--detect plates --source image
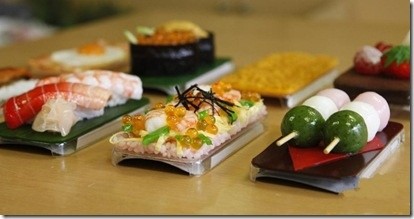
[143,58,236,98]
[249,120,404,178]
[332,66,410,105]
[0,96,150,156]
[263,68,338,110]
[109,116,266,175]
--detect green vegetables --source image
[381,44,410,67]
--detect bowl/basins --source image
[130,31,215,75]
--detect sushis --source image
[0,68,143,137]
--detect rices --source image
[110,96,267,161]
